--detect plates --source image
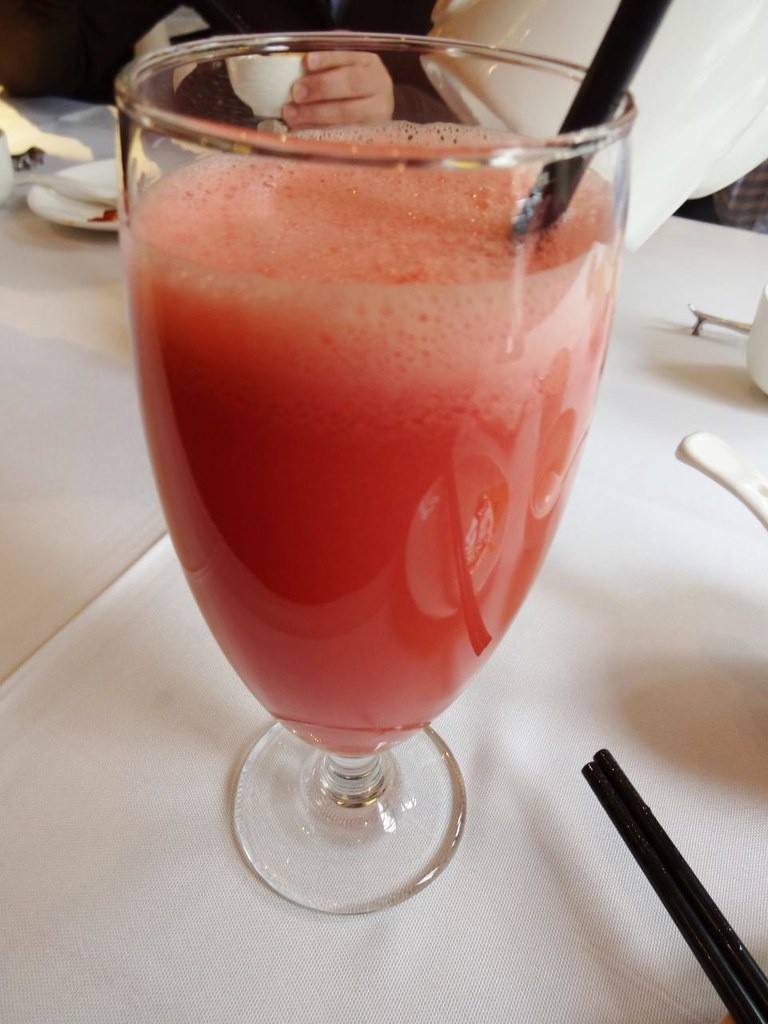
[27,161,118,230]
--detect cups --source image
[745,281,768,395]
[0,128,17,204]
[225,52,306,119]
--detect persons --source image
[0,0,459,131]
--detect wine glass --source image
[117,28,639,917]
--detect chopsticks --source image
[580,747,768,1024]
